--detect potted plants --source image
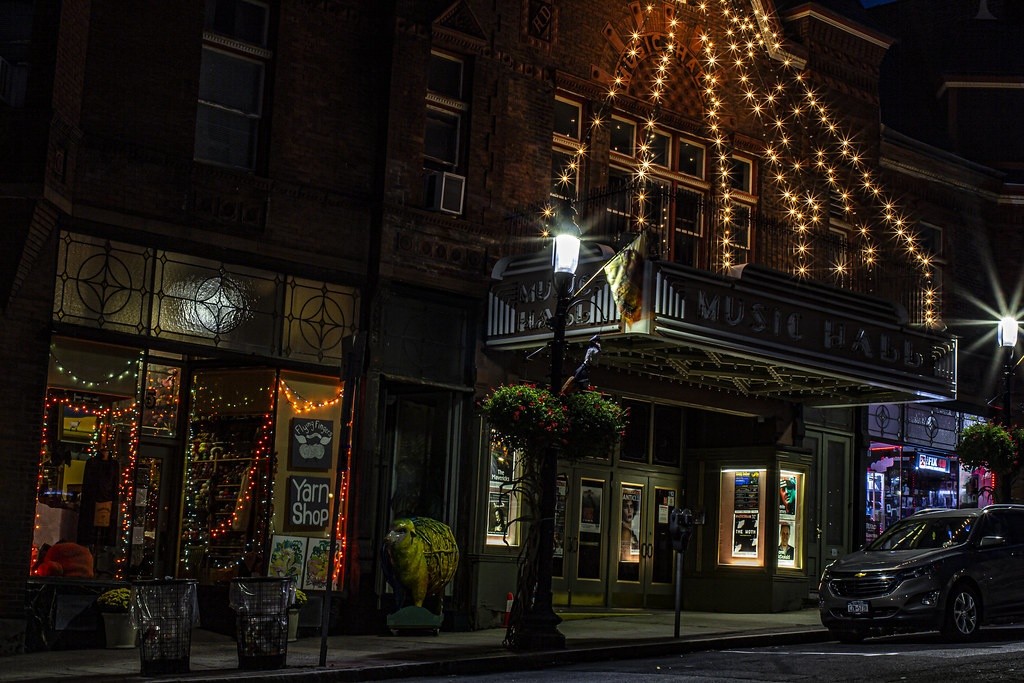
[96,588,139,648]
[289,586,308,642]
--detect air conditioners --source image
[423,171,467,215]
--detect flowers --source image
[956,415,1019,476]
[560,387,633,464]
[473,380,569,455]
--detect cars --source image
[816,503,1024,645]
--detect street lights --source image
[512,190,584,651]
[997,311,1019,504]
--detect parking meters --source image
[667,506,698,640]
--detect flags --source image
[603,234,657,325]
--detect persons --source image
[779,479,795,515]
[620,489,640,561]
[778,522,794,560]
[32,538,67,571]
[490,440,513,484]
[489,507,507,532]
[553,531,564,553]
[583,490,597,523]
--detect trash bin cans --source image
[229,576,291,669]
[132,579,200,676]
